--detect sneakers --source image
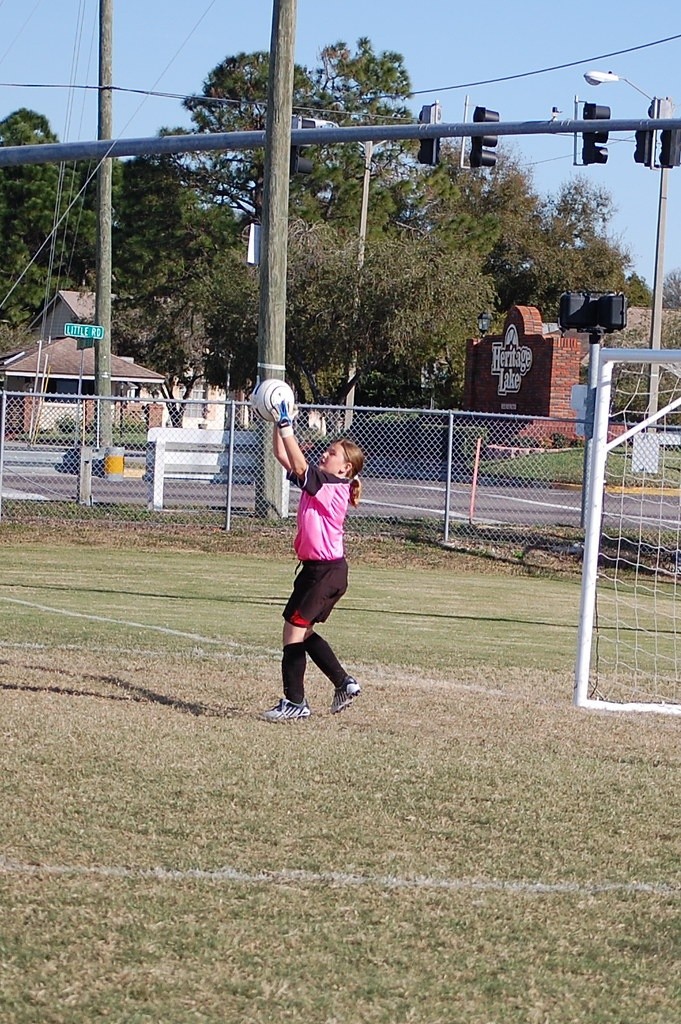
[263,697,310,719]
[331,676,361,715]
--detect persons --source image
[261,400,364,721]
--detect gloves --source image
[270,400,299,438]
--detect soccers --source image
[249,378,296,422]
